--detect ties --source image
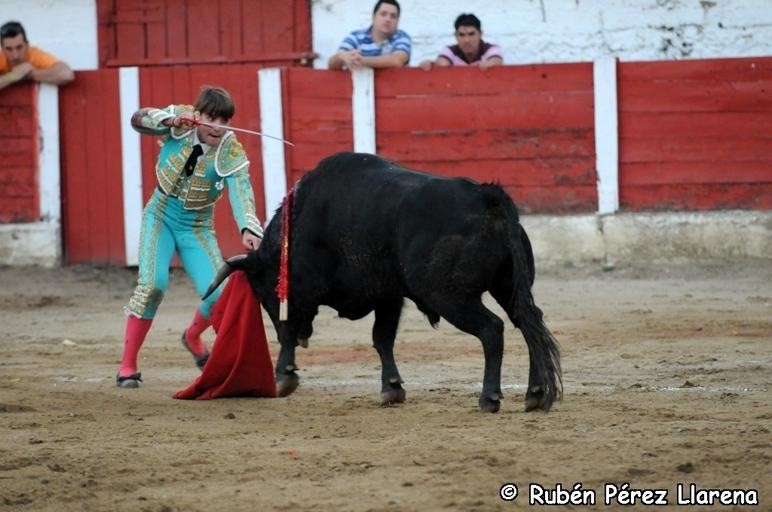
[183,144,206,177]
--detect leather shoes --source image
[180,327,210,372]
[116,369,144,389]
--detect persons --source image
[0,20,77,89]
[329,0,412,71]
[114,86,266,389]
[420,13,503,72]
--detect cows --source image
[200,151,563,415]
